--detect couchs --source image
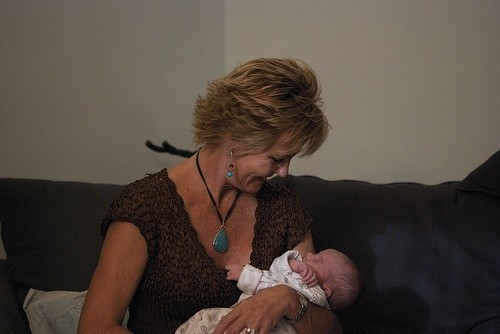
[0,174,500,334]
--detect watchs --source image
[285,292,308,324]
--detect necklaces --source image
[196,147,241,252]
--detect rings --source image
[246,327,255,334]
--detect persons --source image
[77,58,343,334]
[175,249,361,334]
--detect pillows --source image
[457,149,500,199]
[23,288,129,334]
